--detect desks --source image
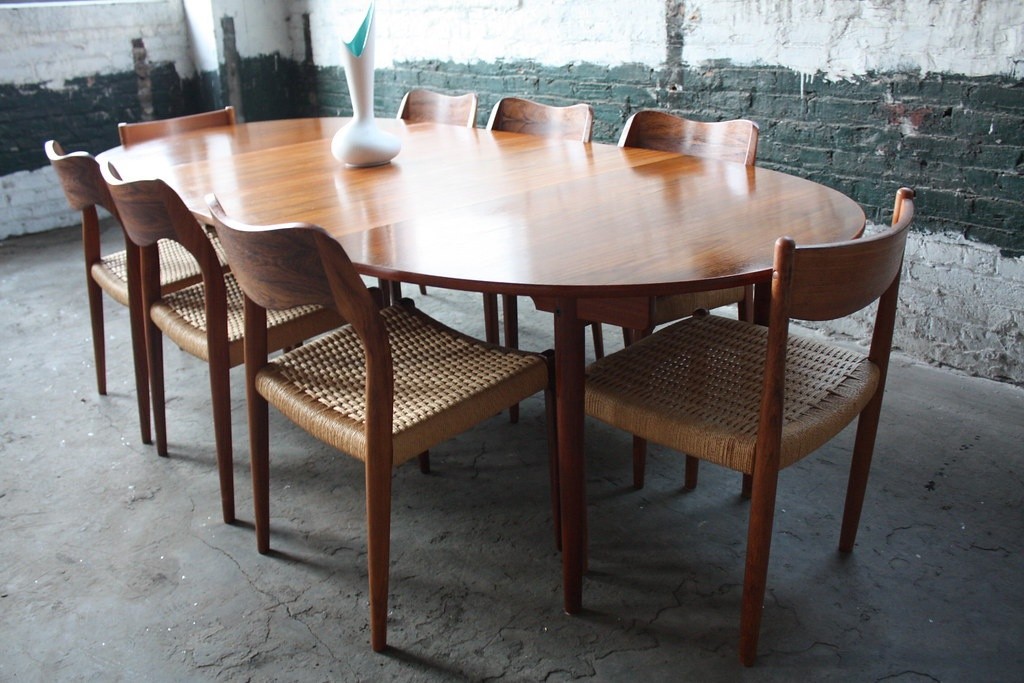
[93,116,868,620]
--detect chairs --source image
[101,160,387,525]
[502,109,759,424]
[397,89,480,129]
[206,191,557,653]
[118,105,236,149]
[44,140,232,446]
[442,96,605,424]
[557,186,918,671]
